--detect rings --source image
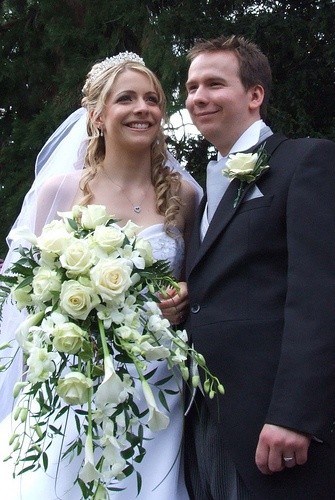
[283,457,294,462]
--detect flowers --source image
[0,203,225,500]
[221,141,270,208]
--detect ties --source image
[206,126,274,224]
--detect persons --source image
[184,34,335,500]
[0,50,203,500]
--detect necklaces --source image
[99,162,152,213]
